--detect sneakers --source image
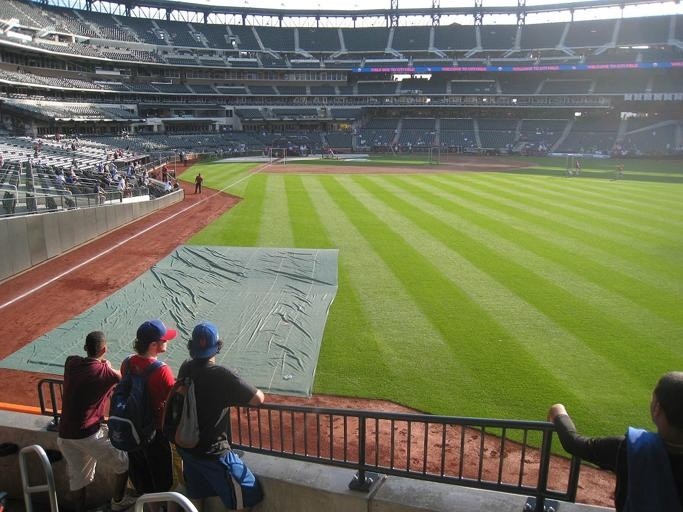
[111,492,139,511]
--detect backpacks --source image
[107,357,165,454]
[162,363,213,449]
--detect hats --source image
[136,318,177,342]
[189,323,220,361]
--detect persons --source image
[173,322,265,510]
[26,117,247,206]
[548,370,683,510]
[55,330,131,509]
[116,319,179,509]
